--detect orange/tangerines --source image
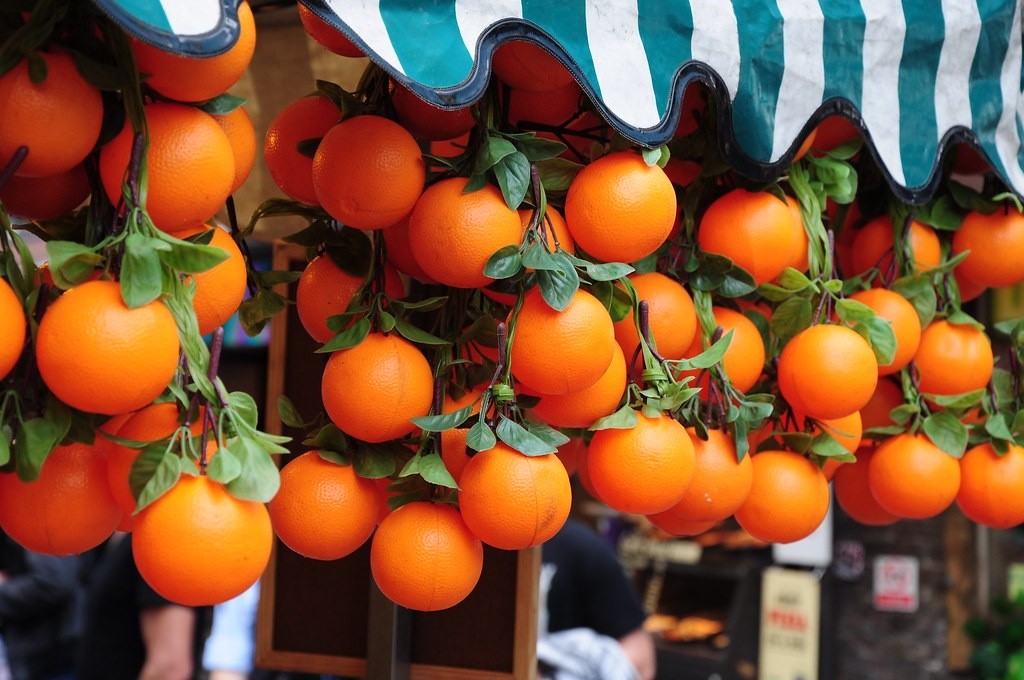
[0,2,1024,612]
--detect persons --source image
[0,530,259,680]
[538,516,656,680]
